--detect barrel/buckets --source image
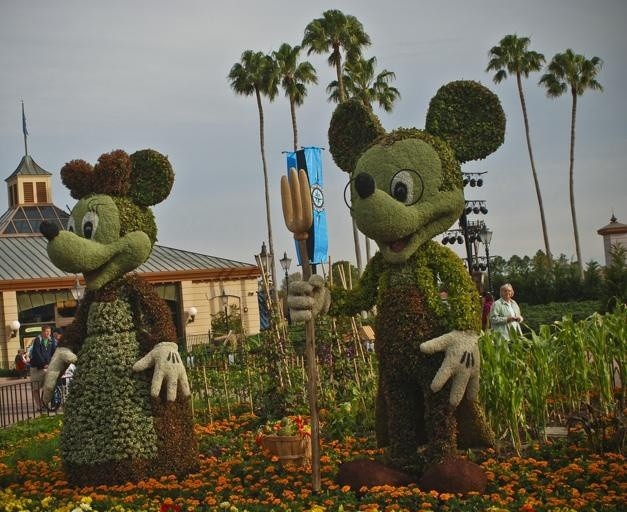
[260,436,276,456]
[276,437,306,469]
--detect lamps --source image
[188,307,196,322]
[10,321,20,337]
[442,173,487,273]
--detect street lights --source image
[279,250,292,326]
[69,273,86,310]
[439,171,493,290]
[479,222,494,301]
[219,290,229,336]
[253,240,276,330]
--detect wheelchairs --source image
[38,361,73,412]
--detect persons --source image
[481,281,524,346]
[14,326,76,412]
